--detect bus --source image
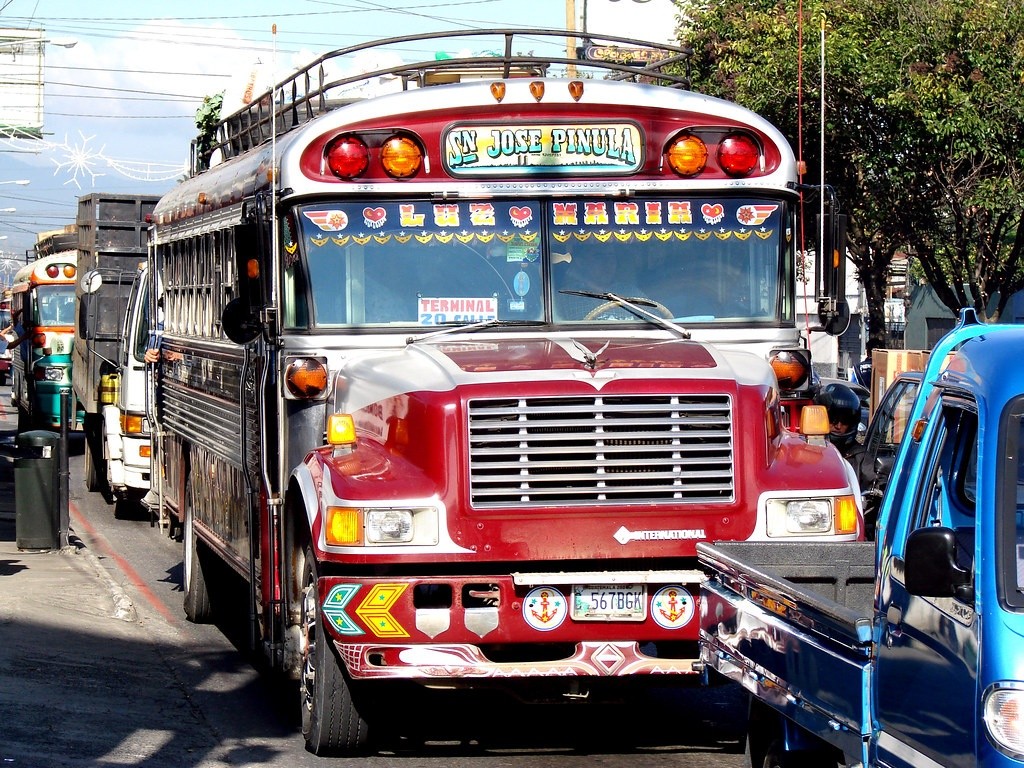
[140,21,872,757]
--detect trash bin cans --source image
[0,429,61,551]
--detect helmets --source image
[800,376,820,399]
[817,383,861,445]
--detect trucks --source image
[690,307,1024,768]
[73,191,159,521]
[0,225,86,448]
[791,250,909,339]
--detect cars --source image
[847,372,1024,540]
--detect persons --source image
[855,338,886,388]
[0,308,34,401]
[814,383,888,542]
[140,292,166,510]
[555,242,661,321]
